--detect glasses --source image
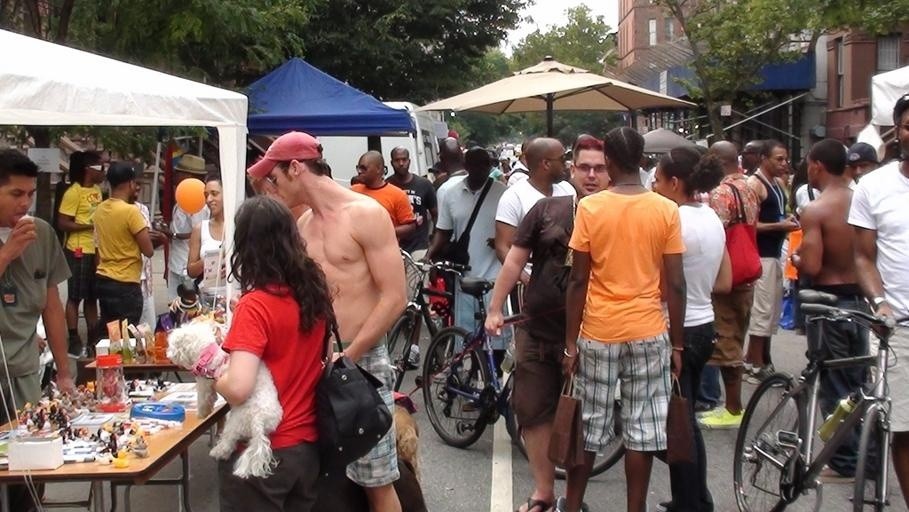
[573,161,609,175]
[355,162,378,171]
[898,122,909,131]
[86,164,106,174]
[549,155,568,164]
[766,155,789,163]
[740,149,758,156]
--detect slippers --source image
[515,493,558,512]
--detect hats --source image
[245,130,327,184]
[845,140,881,167]
[106,159,145,186]
[891,93,909,120]
[171,151,212,177]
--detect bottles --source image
[818,391,860,441]
[500,341,516,373]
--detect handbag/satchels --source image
[654,370,700,470]
[720,180,765,292]
[429,236,473,276]
[313,281,398,469]
[544,369,588,471]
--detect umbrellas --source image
[409,55,699,136]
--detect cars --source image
[501,140,521,156]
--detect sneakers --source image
[551,497,592,512]
[696,404,749,431]
[741,360,754,383]
[814,462,857,485]
[403,343,423,370]
[746,361,795,388]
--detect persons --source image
[0,94,908,510]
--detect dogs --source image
[165,319,284,480]
[394,403,422,487]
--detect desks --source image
[84,327,220,449]
[0,377,229,511]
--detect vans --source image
[307,101,451,204]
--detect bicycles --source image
[733,286,909,512]
[384,246,471,401]
[413,270,627,481]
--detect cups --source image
[94,354,129,413]
[196,375,217,418]
